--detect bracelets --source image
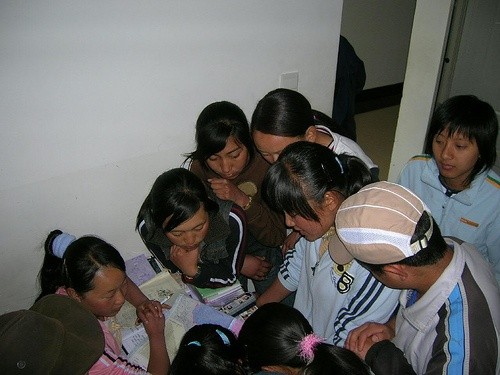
[241,195,253,209]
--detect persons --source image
[250,88,381,183]
[238,302,375,375]
[180,100,296,307]
[335,181,500,375]
[331,35,366,144]
[394,95,500,284]
[28,230,171,375]
[169,324,249,375]
[135,168,248,289]
[253,140,405,347]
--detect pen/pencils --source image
[135,293,174,326]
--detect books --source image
[115,268,259,370]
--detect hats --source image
[1,295,105,375]
[328,181,433,265]
